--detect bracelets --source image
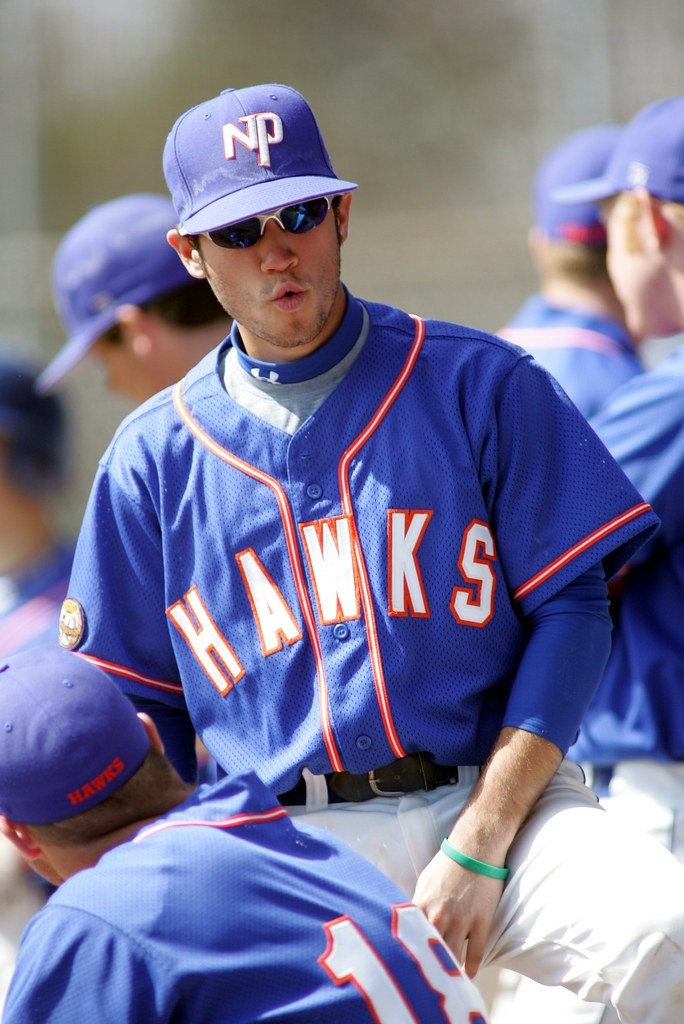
[441,838,508,878]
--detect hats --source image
[161,83,357,235]
[35,193,204,397]
[0,368,63,450]
[0,644,149,825]
[533,123,629,248]
[552,97,684,205]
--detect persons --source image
[61,82,683,1024]
[0,104,684,1024]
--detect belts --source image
[277,750,481,808]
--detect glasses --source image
[178,194,337,249]
